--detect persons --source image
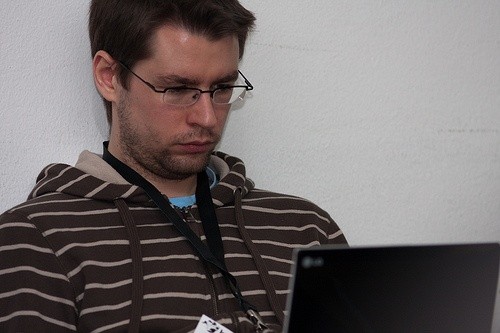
[0,0,351,333]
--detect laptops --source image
[282,242,500,333]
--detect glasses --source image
[115,60,254,106]
